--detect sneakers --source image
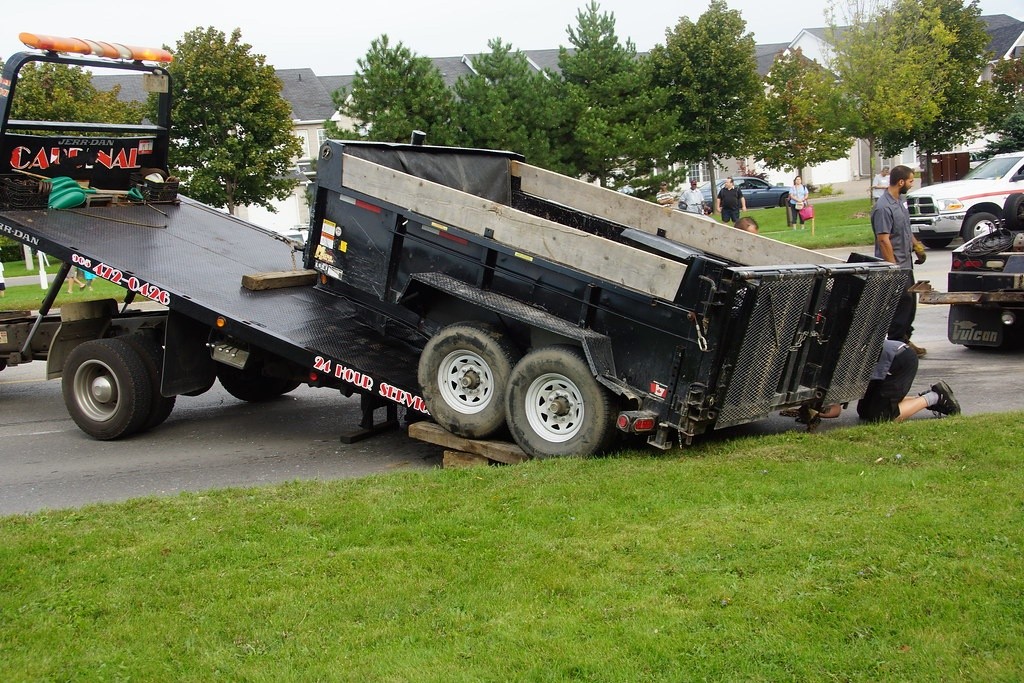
[918,389,949,417]
[929,380,961,415]
[907,340,927,356]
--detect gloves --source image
[912,241,926,264]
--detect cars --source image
[697,177,792,212]
[906,151,1024,250]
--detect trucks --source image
[905,192,1024,351]
[0,32,430,440]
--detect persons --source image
[786,176,809,230]
[656,180,712,216]
[734,217,758,235]
[819,340,961,422]
[65,265,99,293]
[0,261,6,298]
[716,177,746,225]
[871,165,926,357]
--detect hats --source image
[660,181,667,186]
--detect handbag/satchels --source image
[799,199,814,221]
[795,200,804,210]
